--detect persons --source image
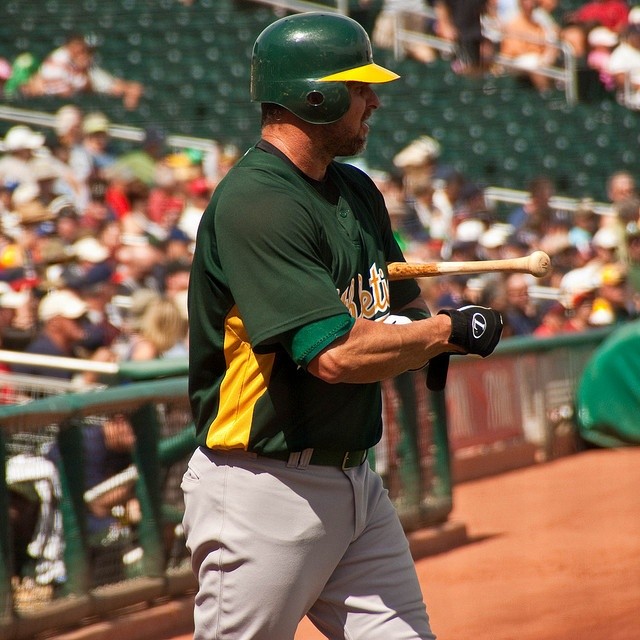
[370,0,640,110]
[374,135,638,344]
[31,32,142,108]
[180,11,505,640]
[0,106,243,395]
[44,413,142,552]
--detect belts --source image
[260,448,370,468]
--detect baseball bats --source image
[384,250,550,281]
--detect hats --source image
[68,26,100,47]
[0,280,30,308]
[75,236,112,263]
[144,127,170,146]
[82,111,109,135]
[3,125,46,152]
[457,219,485,242]
[588,26,620,46]
[188,178,212,196]
[541,233,578,258]
[61,259,117,285]
[38,288,87,321]
[592,229,619,248]
[16,202,58,223]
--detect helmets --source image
[251,12,401,124]
[559,269,601,307]
